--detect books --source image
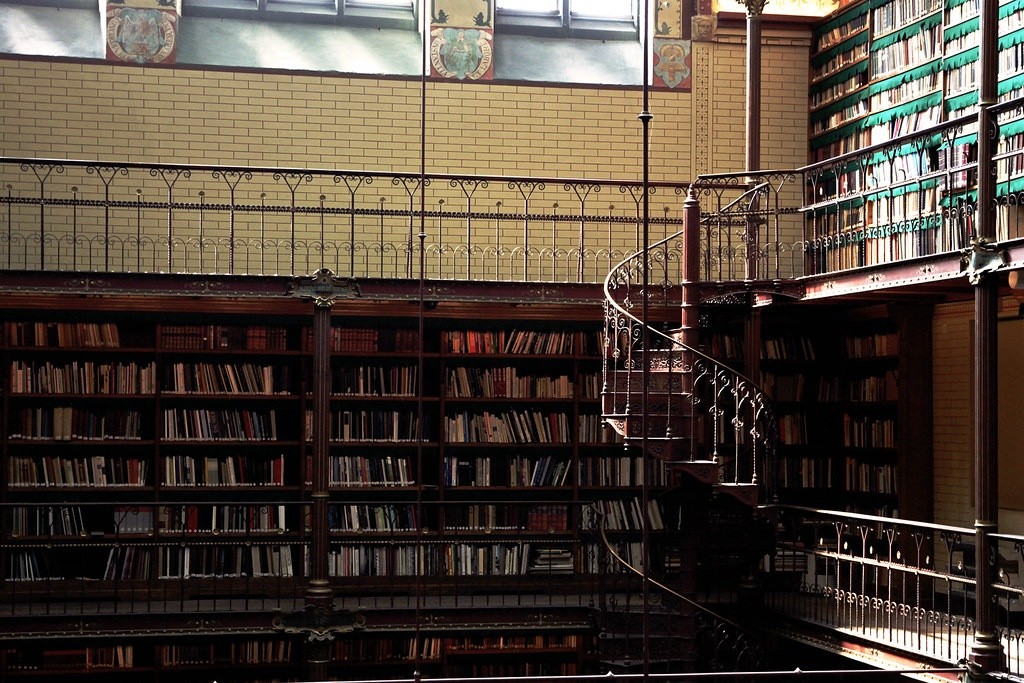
[812,0,1024,272]
[0,322,900,683]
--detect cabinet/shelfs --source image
[801,0,1024,274]
[0,293,937,683]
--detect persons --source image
[694,17,713,40]
